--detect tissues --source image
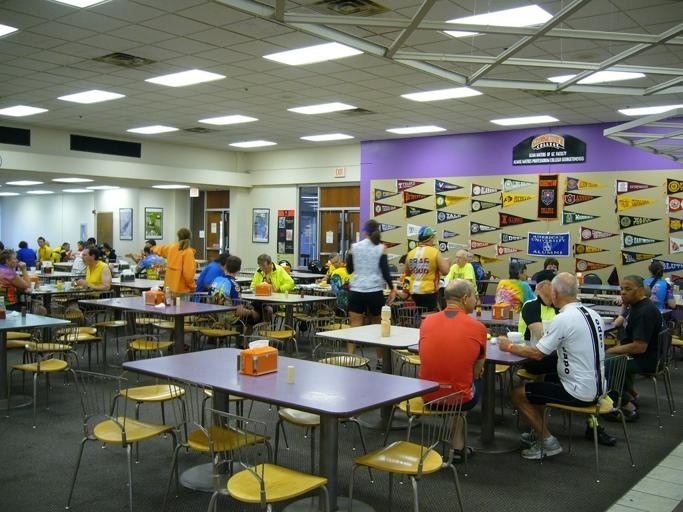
[576,272,584,285]
[255,282,272,296]
[145,286,165,305]
[665,278,674,287]
[240,340,279,376]
[27,275,40,289]
[491,303,511,320]
[40,261,54,273]
[120,269,135,282]
[118,259,129,270]
[280,262,290,274]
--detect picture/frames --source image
[119,208,133,240]
[145,207,163,240]
[252,208,270,243]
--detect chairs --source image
[100,335,190,465]
[65,368,180,511]
[2,260,683,430]
[537,355,636,485]
[164,374,274,512]
[202,404,331,511]
[346,389,466,512]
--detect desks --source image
[121,346,441,511]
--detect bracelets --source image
[508,344,513,353]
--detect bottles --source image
[283,289,305,298]
[380,303,391,337]
[286,365,295,383]
[476,299,481,318]
[0,296,6,319]
[165,287,171,305]
[175,296,180,306]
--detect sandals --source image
[453,446,476,464]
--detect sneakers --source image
[520,429,538,444]
[600,409,637,423]
[520,437,562,460]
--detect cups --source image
[673,295,682,303]
[27,260,80,289]
[321,280,326,287]
[506,331,522,343]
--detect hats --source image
[536,270,556,284]
[418,227,436,241]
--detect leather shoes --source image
[586,425,617,446]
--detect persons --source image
[517,271,639,447]
[190,253,260,348]
[418,278,487,463]
[531,258,559,292]
[1,236,117,313]
[498,271,607,461]
[494,262,537,314]
[605,261,677,414]
[314,251,354,332]
[135,240,166,280]
[250,253,295,327]
[150,228,198,294]
[345,219,397,370]
[396,225,485,313]
